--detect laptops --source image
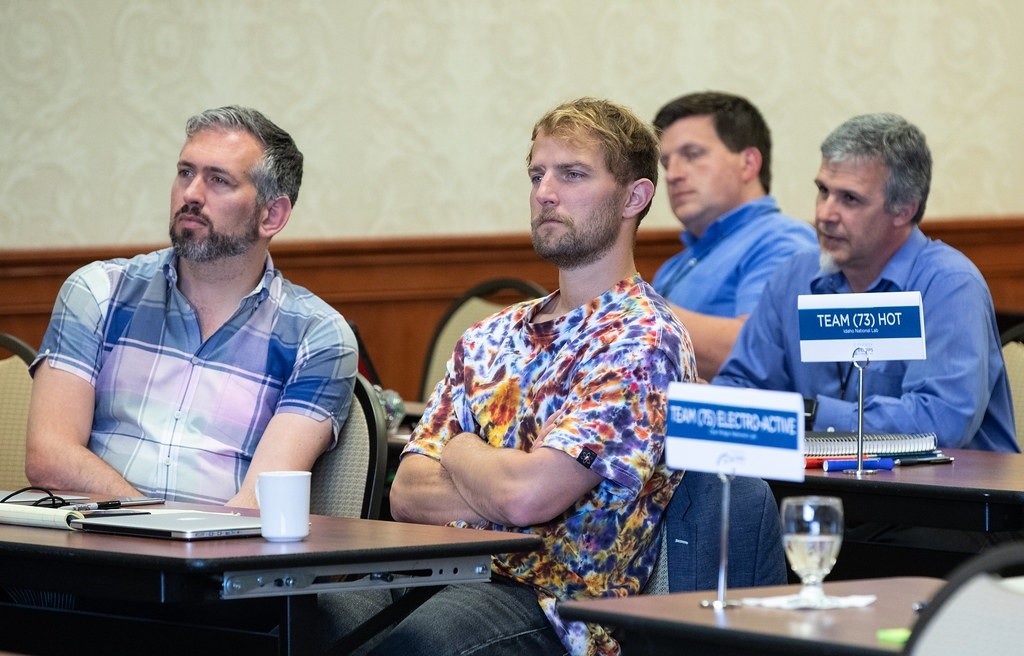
[68,510,263,541]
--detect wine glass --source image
[778,495,842,607]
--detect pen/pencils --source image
[911,600,928,613]
[73,500,121,511]
[894,457,955,466]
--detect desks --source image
[768,447,1024,582]
[0,489,546,656]
[557,577,947,656]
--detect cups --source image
[254,471,311,543]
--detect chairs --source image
[1001,313,1024,452]
[309,372,388,520]
[416,275,546,401]
[0,332,38,492]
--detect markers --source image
[805,455,869,469]
[823,458,894,471]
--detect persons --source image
[650,90,818,389]
[23,104,359,510]
[291,96,699,656]
[709,112,1021,455]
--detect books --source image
[803,431,938,457]
